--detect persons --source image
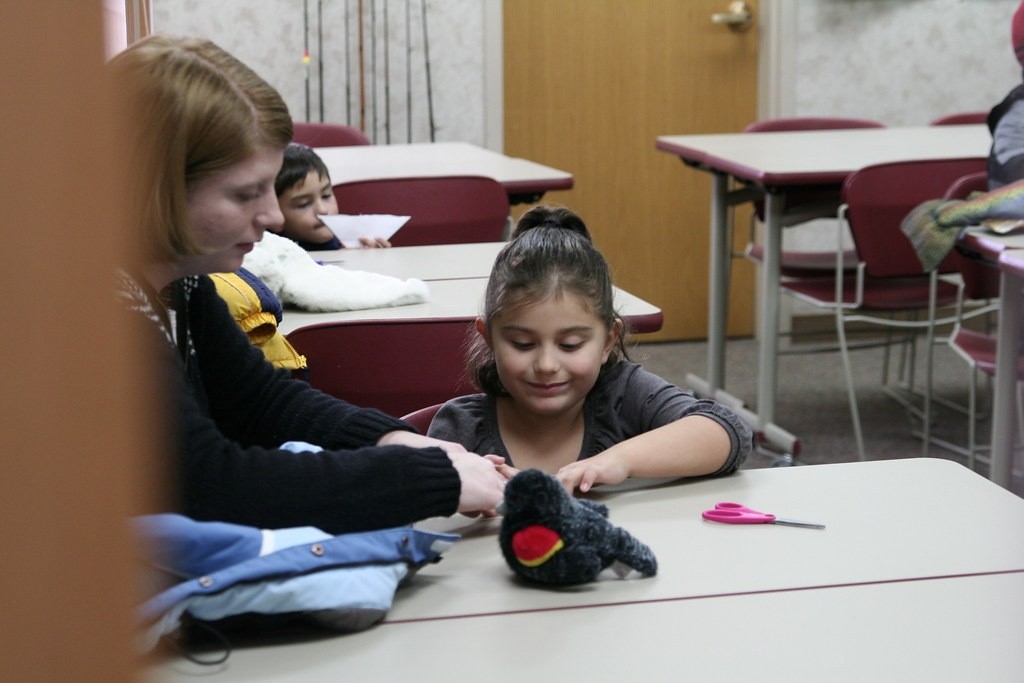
[427,206,753,497]
[986,1,1024,195]
[102,30,509,534]
[274,143,392,252]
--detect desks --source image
[124,113,1023,682]
[310,139,576,206]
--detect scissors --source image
[701,501,826,529]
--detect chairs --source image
[329,177,517,252]
[779,154,991,462]
[915,173,1024,482]
[285,316,481,420]
[750,117,889,286]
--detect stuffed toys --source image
[498,469,658,589]
